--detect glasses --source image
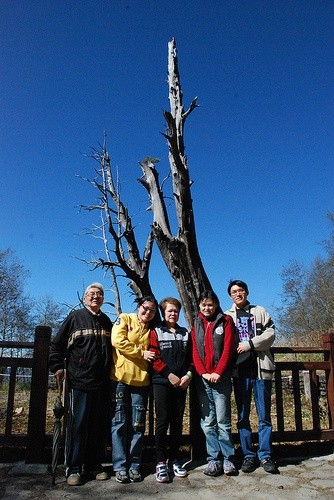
[141,305,156,313]
[85,292,103,299]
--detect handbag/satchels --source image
[236,351,258,378]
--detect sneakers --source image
[155,461,169,483]
[165,458,187,477]
[114,470,141,484]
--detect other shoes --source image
[202,460,222,476]
[67,473,82,486]
[85,469,110,480]
[260,457,274,472]
[241,457,258,473]
[223,459,237,474]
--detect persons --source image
[222,279,278,473]
[47,281,112,485]
[188,290,238,476]
[148,297,192,483]
[111,297,158,484]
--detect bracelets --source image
[186,374,192,380]
